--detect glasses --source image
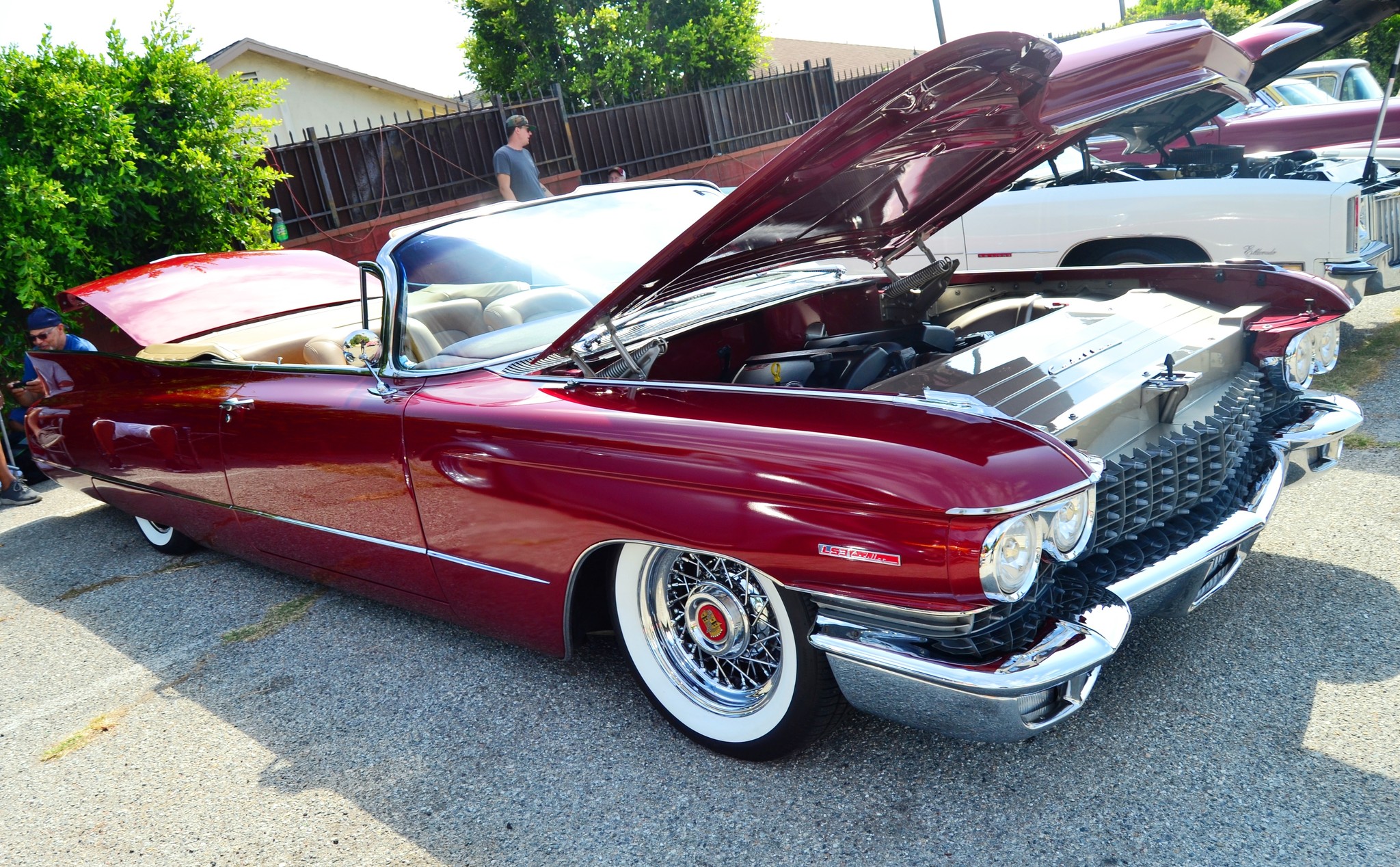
[519,127,530,132]
[29,327,56,341]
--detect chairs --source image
[302,284,595,369]
[96,418,210,469]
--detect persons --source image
[0,392,43,505]
[606,167,626,182]
[7,308,97,433]
[493,115,554,202]
[359,337,366,354]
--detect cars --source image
[885,0,1400,309]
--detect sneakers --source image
[0,478,44,505]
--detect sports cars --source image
[21,27,1363,764]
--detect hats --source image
[506,115,537,131]
[28,307,70,330]
[608,166,626,179]
